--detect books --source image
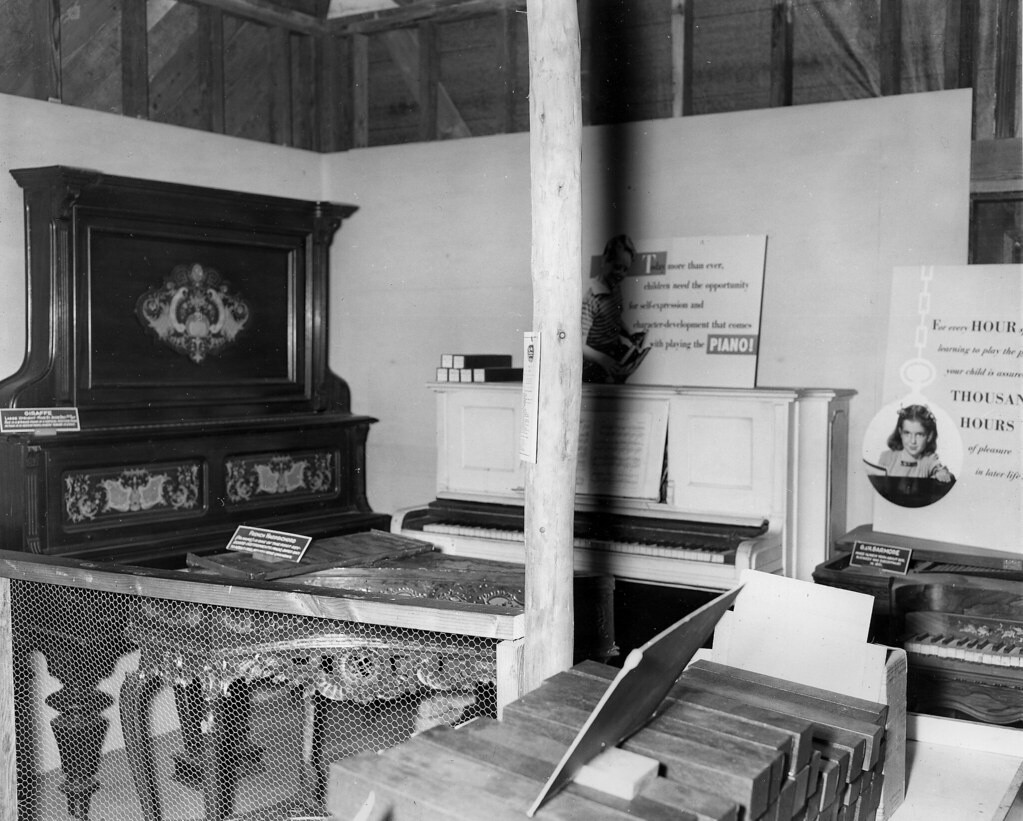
[578,395,670,502]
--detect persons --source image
[580,233,637,384]
[878,402,954,484]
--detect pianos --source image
[811,520,1023,729]
[387,377,859,599]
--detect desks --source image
[118,556,619,821]
